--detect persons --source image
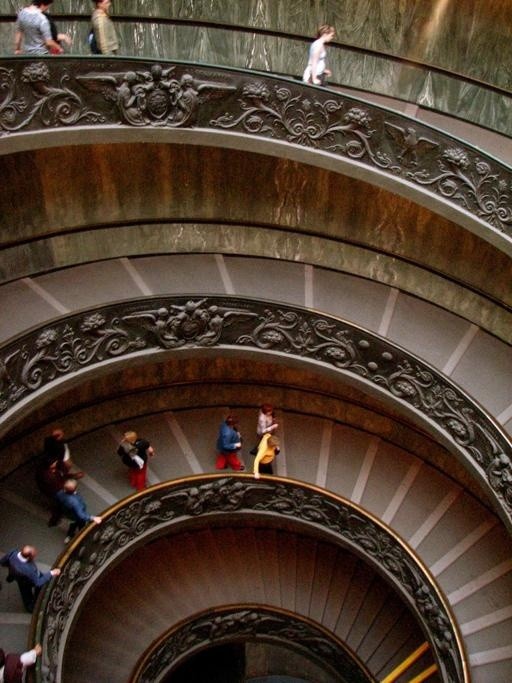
[14,0,66,54]
[249,402,281,456]
[302,24,335,88]
[33,449,85,527]
[215,414,245,470]
[43,429,85,478]
[57,478,103,545]
[1,545,62,614]
[252,433,282,479]
[31,0,76,53]
[91,0,121,55]
[0,641,43,683]
[116,430,156,491]
